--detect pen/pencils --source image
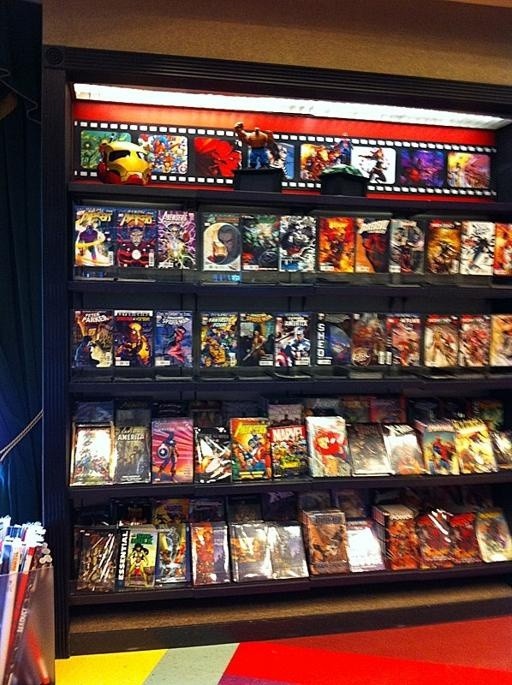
[0,517,56,685]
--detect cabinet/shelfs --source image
[41,49,511,657]
